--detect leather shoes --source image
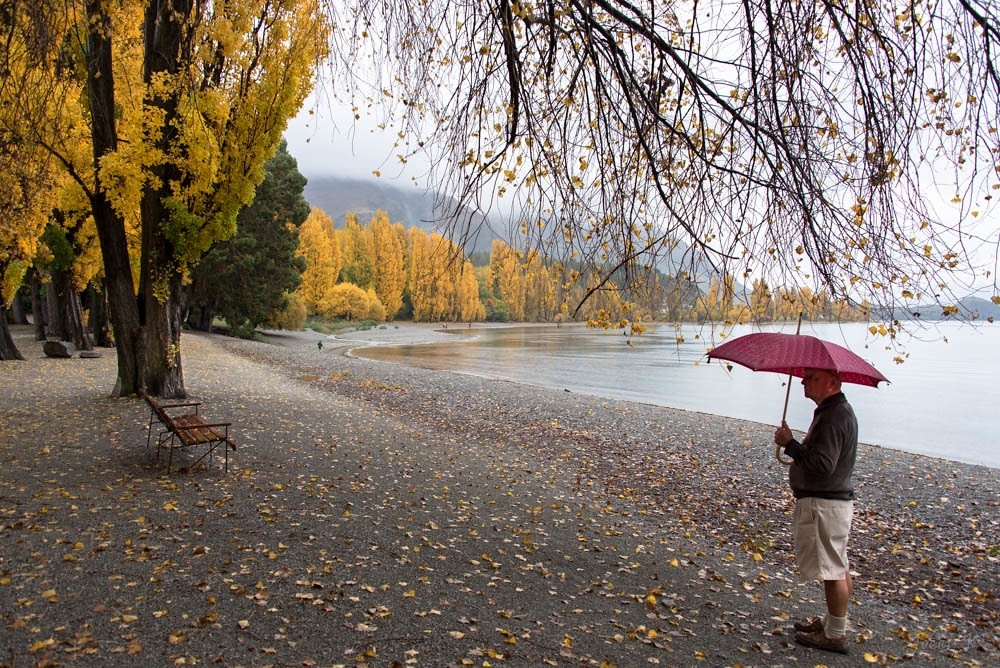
[795,629,849,655]
[794,617,824,633]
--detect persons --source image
[774,365,860,655]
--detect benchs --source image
[139,388,238,475]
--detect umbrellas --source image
[703,311,892,465]
